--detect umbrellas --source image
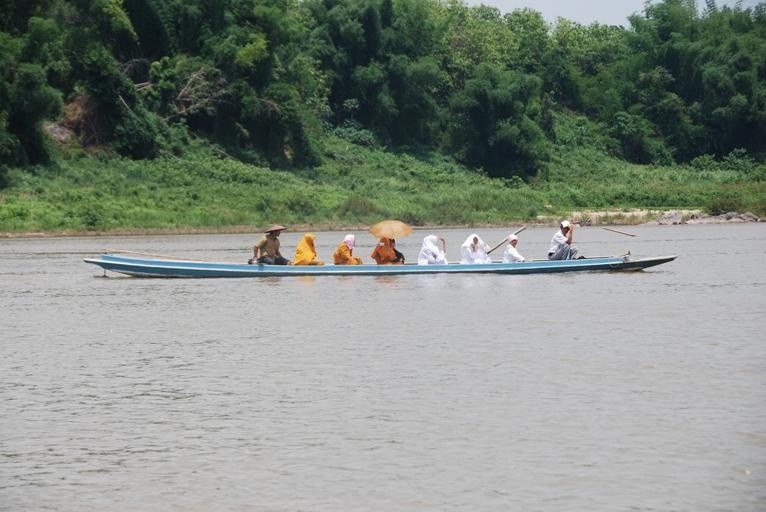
[369,218,413,238]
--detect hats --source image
[560,220,571,228]
[265,224,287,233]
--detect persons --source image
[391,238,405,264]
[253,224,292,265]
[461,234,492,264]
[418,235,448,264]
[332,234,362,264]
[370,236,402,264]
[503,234,528,263]
[293,234,324,265]
[546,220,579,259]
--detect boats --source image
[85,253,678,280]
